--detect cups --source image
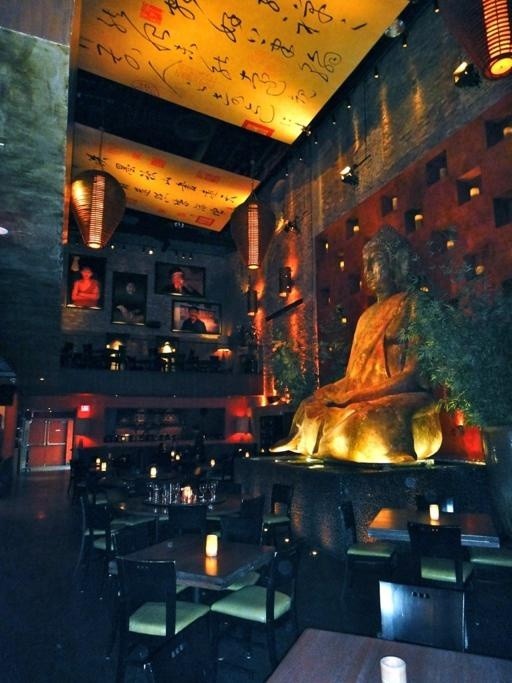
[380,656,407,683]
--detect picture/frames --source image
[170,299,222,334]
[66,253,108,310]
[155,261,206,298]
[110,271,148,325]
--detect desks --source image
[262,625,510,683]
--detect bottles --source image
[145,481,181,506]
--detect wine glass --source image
[198,480,219,504]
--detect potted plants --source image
[364,223,511,551]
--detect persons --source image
[285,223,456,464]
[181,306,208,332]
[173,269,185,292]
[116,281,141,324]
[191,424,205,463]
[71,268,100,307]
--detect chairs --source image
[112,554,211,683]
[68,448,298,603]
[104,519,160,657]
[205,537,311,668]
[374,578,471,652]
[332,495,512,619]
[69,342,255,375]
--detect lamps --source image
[72,127,128,249]
[480,0,512,80]
[452,60,479,89]
[284,0,441,177]
[246,157,261,271]
[74,235,193,260]
[247,291,257,316]
[273,217,300,236]
[278,266,291,298]
[338,165,359,188]
